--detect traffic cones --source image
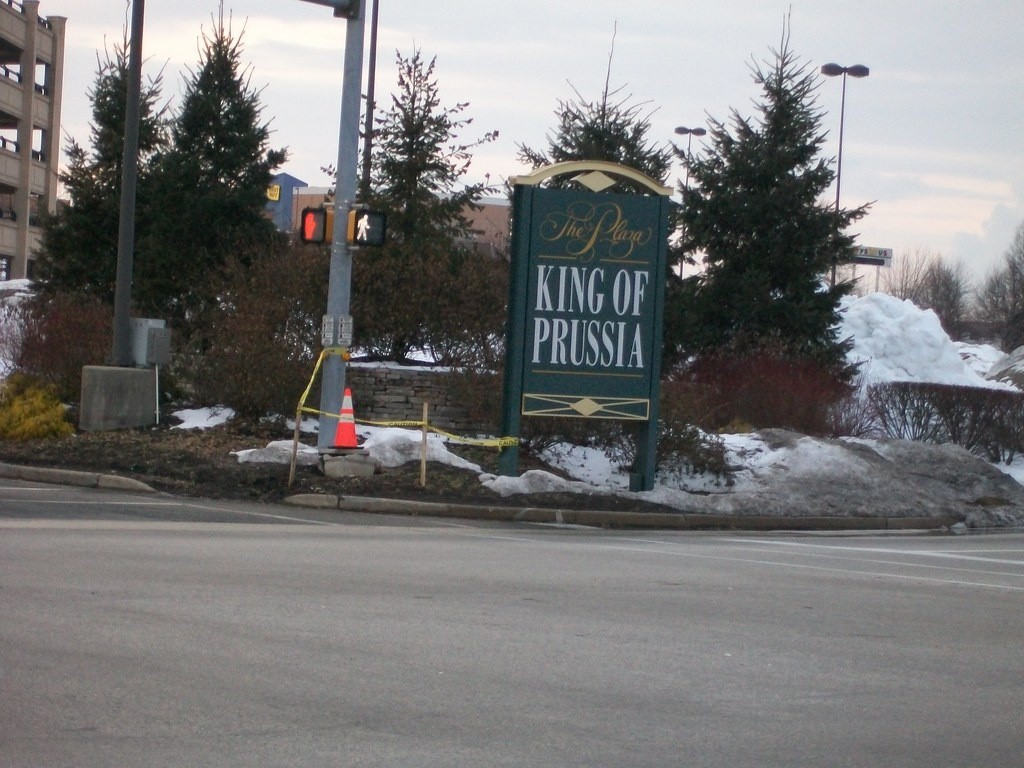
[328,388,365,449]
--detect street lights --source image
[673,125,707,279]
[820,62,870,292]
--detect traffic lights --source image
[299,206,334,245]
[347,208,387,249]
